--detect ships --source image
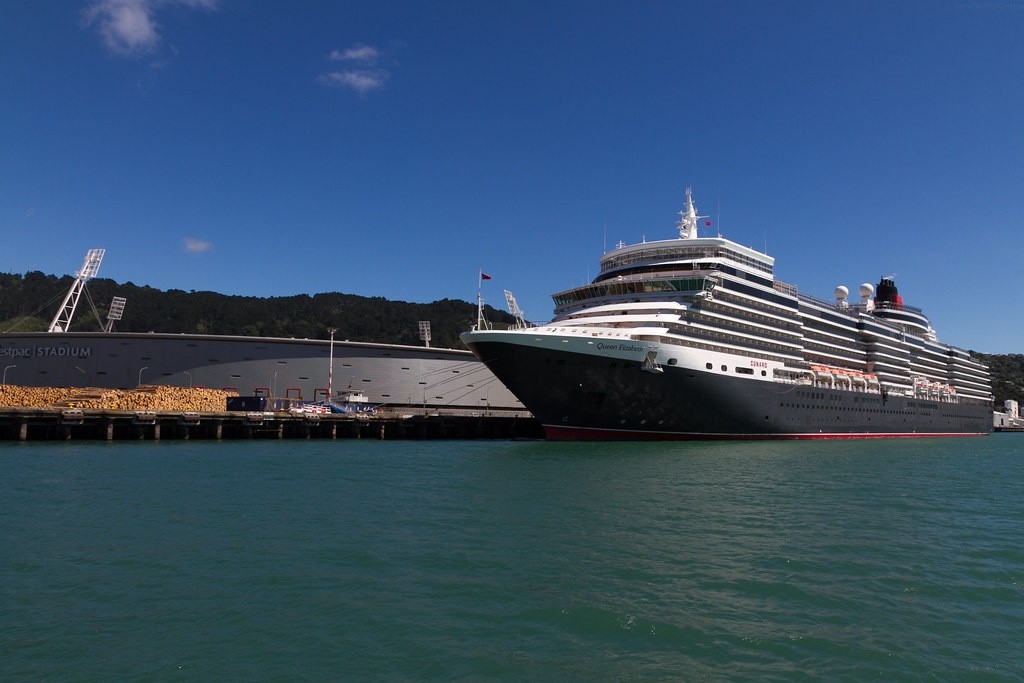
[459,184,995,441]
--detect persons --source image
[774,368,805,380]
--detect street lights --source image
[3,365,17,385]
[328,327,337,401]
[139,367,148,387]
[184,372,192,389]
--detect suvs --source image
[363,409,376,415]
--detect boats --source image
[917,387,956,398]
[814,371,878,388]
